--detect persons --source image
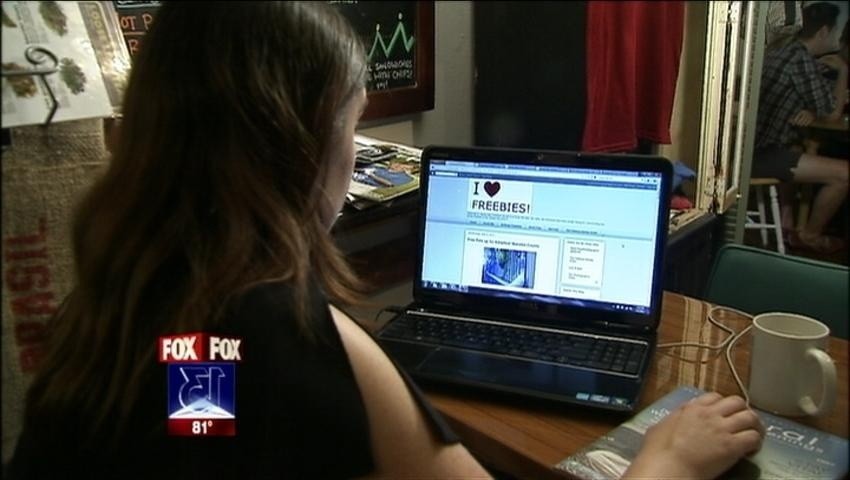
[4,0,767,480]
[750,2,850,255]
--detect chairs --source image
[702,241,850,340]
[723,100,786,255]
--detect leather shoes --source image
[782,226,844,253]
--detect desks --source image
[340,232,849,478]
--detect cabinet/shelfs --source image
[470,0,652,158]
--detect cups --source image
[748,310,838,419]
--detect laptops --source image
[375,144,675,417]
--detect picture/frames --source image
[331,0,435,128]
